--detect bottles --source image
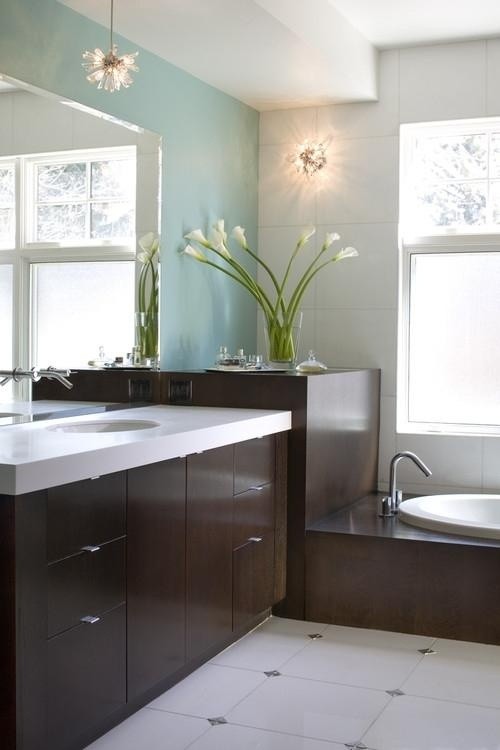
[215,346,245,367]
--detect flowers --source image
[132,231,158,360]
[178,213,361,361]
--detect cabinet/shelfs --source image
[33,470,128,750]
[235,429,276,633]
[127,442,235,705]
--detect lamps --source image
[288,136,328,178]
[80,0,141,94]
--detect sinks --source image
[0,410,23,419]
[44,415,161,435]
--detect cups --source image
[249,354,263,368]
[133,346,141,366]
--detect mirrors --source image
[0,72,165,428]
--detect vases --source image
[263,312,305,373]
[132,312,158,369]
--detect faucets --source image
[378,449,432,518]
[32,367,74,391]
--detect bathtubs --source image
[397,492,500,538]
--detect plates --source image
[85,360,151,370]
[203,364,290,373]
